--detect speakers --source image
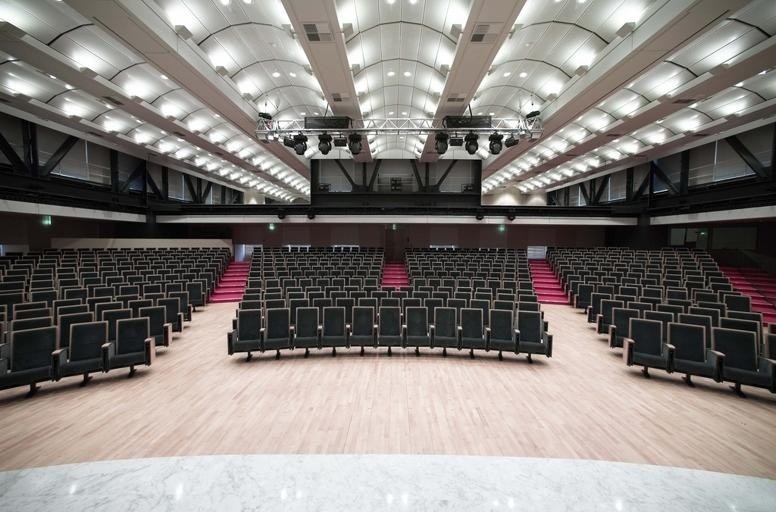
[445,114,491,129]
[304,116,349,129]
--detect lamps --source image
[435,131,449,154]
[488,131,502,155]
[318,131,332,155]
[293,134,308,155]
[348,131,363,154]
[464,130,480,155]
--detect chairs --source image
[442,279,456,291]
[503,280,516,290]
[609,307,640,350]
[101,309,134,344]
[286,286,305,293]
[263,293,284,300]
[324,286,340,298]
[573,246,637,286]
[119,286,142,300]
[10,315,54,334]
[363,286,378,299]
[247,280,264,291]
[94,301,124,323]
[371,290,390,299]
[666,290,688,300]
[698,300,728,318]
[381,286,395,298]
[688,307,719,327]
[639,297,661,311]
[357,298,379,324]
[113,316,155,378]
[286,292,305,305]
[380,297,403,331]
[493,300,515,310]
[86,296,112,312]
[573,284,592,308]
[722,294,751,313]
[143,284,163,295]
[623,318,666,378]
[428,279,441,292]
[244,293,264,301]
[30,290,58,308]
[0,293,23,318]
[169,291,192,322]
[336,298,352,330]
[137,305,173,355]
[0,326,64,399]
[315,279,329,291]
[473,280,486,288]
[157,298,185,332]
[615,295,636,309]
[667,300,694,314]
[676,313,713,357]
[399,286,413,298]
[115,295,138,309]
[619,286,639,301]
[596,299,624,333]
[65,320,112,387]
[84,245,207,287]
[207,247,232,300]
[475,287,494,294]
[364,278,378,288]
[486,309,514,360]
[401,247,415,285]
[656,304,684,314]
[266,287,282,293]
[144,293,165,306]
[446,298,465,326]
[349,278,359,290]
[374,307,402,357]
[640,288,662,299]
[350,291,367,298]
[637,244,707,291]
[719,317,761,363]
[596,285,615,301]
[454,292,472,308]
[416,247,471,279]
[331,278,345,291]
[430,307,458,358]
[495,288,515,294]
[437,286,453,299]
[693,292,719,303]
[643,310,673,353]
[259,308,290,360]
[127,298,154,319]
[515,311,552,364]
[516,289,535,295]
[378,246,385,287]
[64,289,88,304]
[497,293,516,301]
[186,282,207,313]
[391,291,408,299]
[424,298,444,324]
[401,307,430,356]
[290,307,319,359]
[238,300,263,310]
[487,280,500,292]
[458,279,471,288]
[667,322,715,386]
[15,307,52,320]
[708,252,740,294]
[471,249,533,280]
[456,287,473,292]
[13,301,50,310]
[298,280,312,291]
[282,279,295,292]
[346,307,374,357]
[305,286,322,298]
[264,280,281,288]
[58,310,95,358]
[330,290,348,306]
[518,301,541,312]
[432,291,449,307]
[317,307,346,356]
[458,308,485,359]
[288,299,311,307]
[246,289,265,295]
[248,246,377,280]
[313,298,333,307]
[55,304,90,316]
[53,299,81,327]
[474,292,493,301]
[627,302,653,318]
[543,244,574,304]
[343,286,360,299]
[264,299,286,308]
[587,293,609,323]
[93,287,116,302]
[418,286,434,299]
[403,298,424,325]
[165,283,182,298]
[518,281,533,290]
[227,309,260,363]
[308,292,324,306]
[519,294,538,302]
[713,328,775,396]
[411,291,430,299]
[470,298,490,328]
[727,310,762,344]
[412,279,426,292]
[0,247,85,293]
